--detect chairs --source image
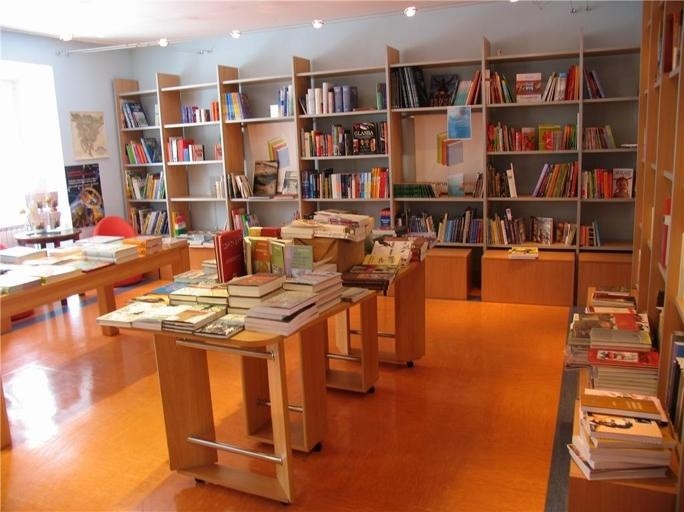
[93,217,143,287]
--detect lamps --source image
[158,7,424,50]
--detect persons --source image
[613,177,630,199]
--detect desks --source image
[14,229,89,307]
[567,284,682,512]
[97,292,379,502]
[0,241,197,345]
[337,259,426,367]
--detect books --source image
[486,122,577,151]
[2,233,186,293]
[660,196,672,271]
[564,280,684,482]
[393,171,484,244]
[167,101,223,162]
[392,66,482,109]
[583,68,605,98]
[222,84,293,122]
[98,226,432,342]
[486,64,579,106]
[118,97,170,238]
[612,168,633,199]
[582,125,618,151]
[228,160,298,236]
[579,215,602,248]
[581,168,613,199]
[298,81,389,199]
[486,162,577,246]
[508,247,538,260]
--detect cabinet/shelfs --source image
[296,46,391,233]
[481,34,579,309]
[114,73,172,237]
[630,1,681,475]
[386,38,479,301]
[219,55,302,227]
[579,25,639,311]
[156,64,231,232]
